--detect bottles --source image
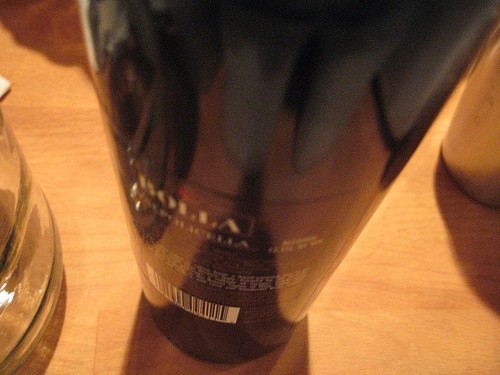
[79,0,500,365]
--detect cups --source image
[0,110,64,375]
[439,0,500,211]
